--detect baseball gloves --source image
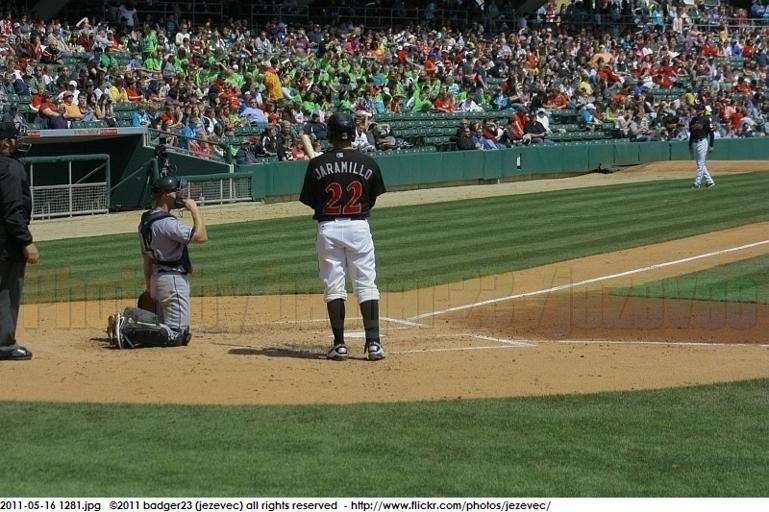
[138,291,156,312]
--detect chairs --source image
[1,49,769,166]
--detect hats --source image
[63,91,75,98]
[43,91,55,96]
[70,80,77,85]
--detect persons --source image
[0,2,768,164]
[299,111,387,363]
[687,102,717,194]
[106,171,210,351]
[1,107,39,360]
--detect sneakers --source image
[367,342,386,361]
[107,313,126,350]
[326,345,347,360]
[0,348,31,360]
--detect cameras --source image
[107,99,112,103]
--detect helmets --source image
[1,122,32,155]
[152,176,188,207]
[327,114,356,141]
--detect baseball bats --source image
[301,134,315,160]
